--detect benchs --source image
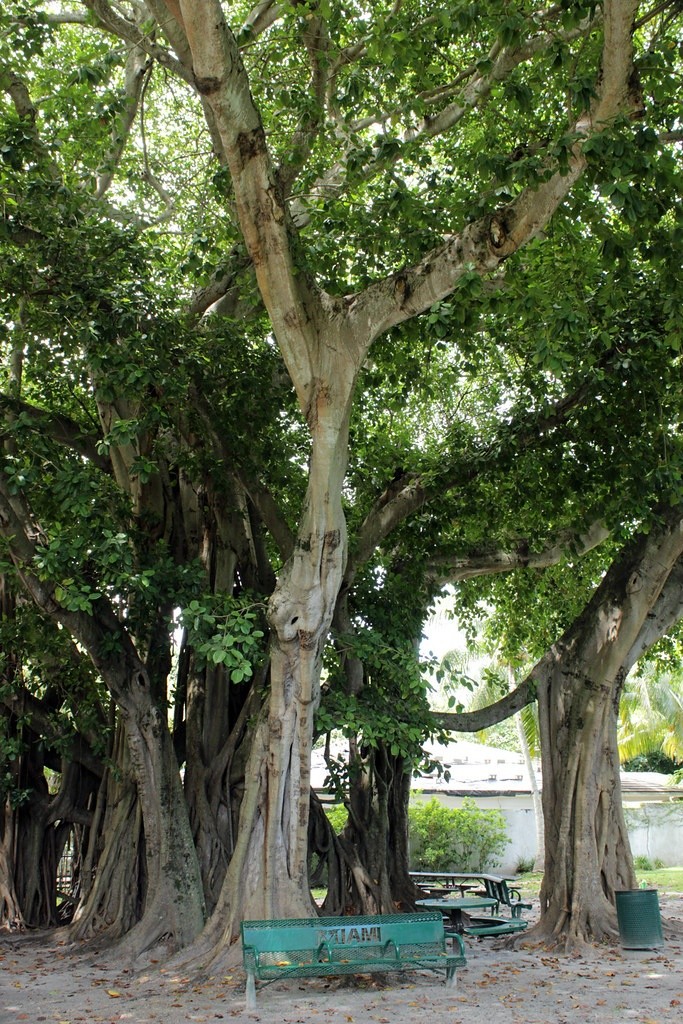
[466,890,487,912]
[422,888,459,897]
[240,911,467,1009]
[481,873,532,918]
[416,884,436,890]
[442,884,480,898]
[442,916,528,936]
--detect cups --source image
[639,883,642,888]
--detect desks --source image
[409,872,522,886]
[415,898,498,909]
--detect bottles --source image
[642,879,646,889]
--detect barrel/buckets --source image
[615,889,664,949]
